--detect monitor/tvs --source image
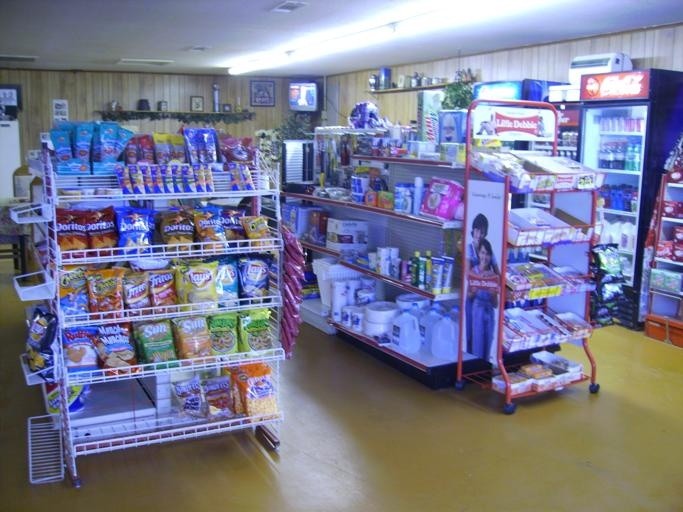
[288,80,320,113]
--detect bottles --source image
[385,256,391,274]
[426,250,432,287]
[411,177,423,215]
[235,98,242,113]
[529,126,577,208]
[595,116,642,279]
[157,101,168,111]
[110,100,119,111]
[407,119,417,142]
[412,249,420,286]
[138,99,151,109]
[369,67,395,90]
[212,82,220,112]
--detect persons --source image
[468,213,499,361]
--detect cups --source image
[411,77,441,86]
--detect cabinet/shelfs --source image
[280,153,464,375]
[645,173,682,348]
[9,149,285,489]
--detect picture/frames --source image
[190,96,204,111]
[0,84,21,112]
[250,81,276,107]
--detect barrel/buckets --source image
[391,301,468,361]
[598,214,637,252]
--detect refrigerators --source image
[530,101,577,155]
[578,66,683,330]
[471,79,568,171]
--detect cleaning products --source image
[413,176,425,217]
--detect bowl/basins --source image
[324,187,347,200]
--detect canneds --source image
[534,131,579,160]
[598,117,645,132]
